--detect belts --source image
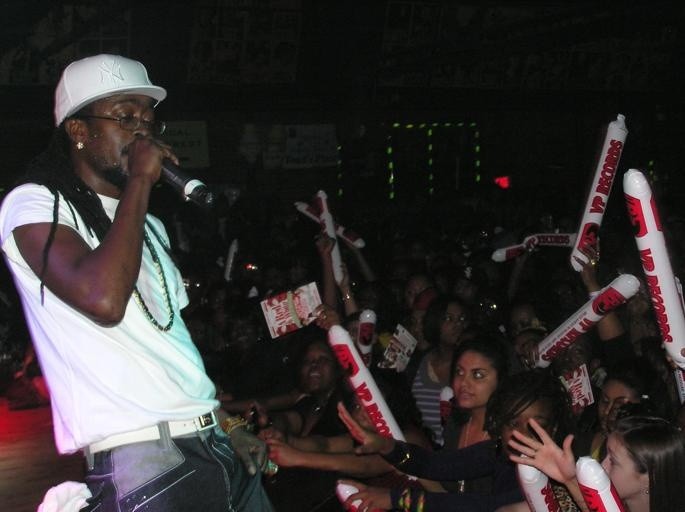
[84,411,217,456]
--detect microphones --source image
[160,157,216,209]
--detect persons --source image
[1,175,685,489]
[0,53,277,512]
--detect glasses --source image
[74,113,167,136]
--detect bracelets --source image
[218,415,249,433]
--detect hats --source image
[54,53,167,128]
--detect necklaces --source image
[134,228,174,331]
